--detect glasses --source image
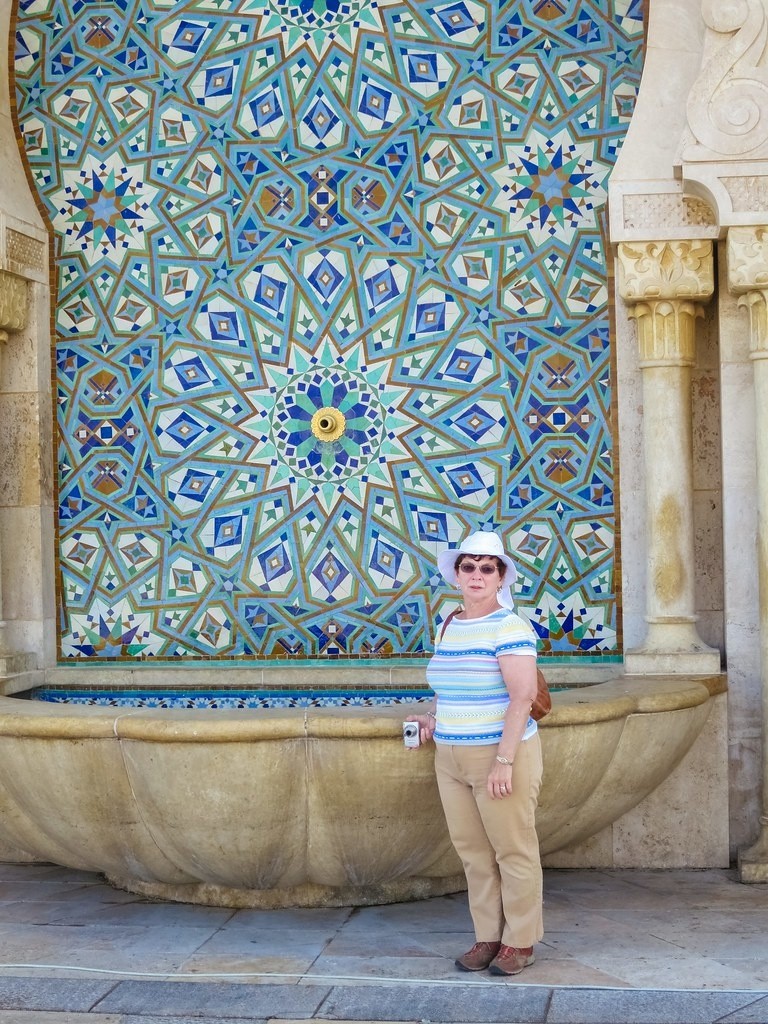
[458,562,499,574]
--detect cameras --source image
[403,722,420,747]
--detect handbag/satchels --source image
[530,666,551,721]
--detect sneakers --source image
[489,944,534,975]
[455,941,501,970]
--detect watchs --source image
[494,754,514,766]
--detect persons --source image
[406,531,544,977]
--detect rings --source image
[500,787,505,789]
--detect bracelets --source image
[426,711,436,720]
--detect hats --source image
[437,531,518,588]
